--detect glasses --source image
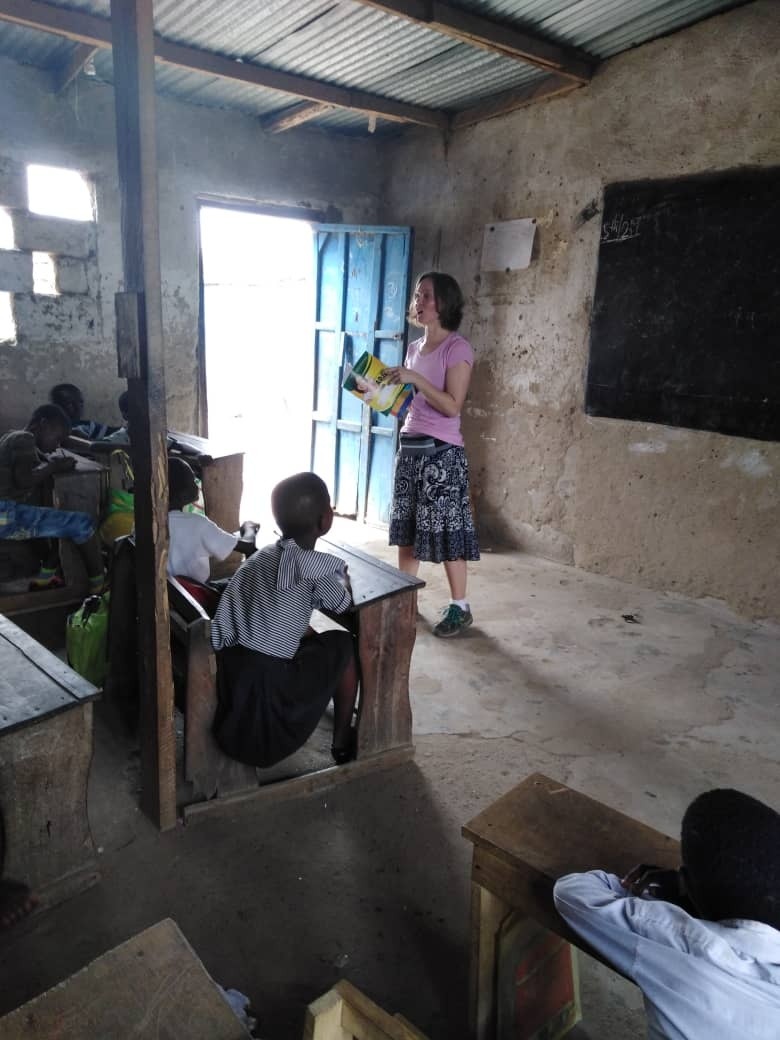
[59,399,84,409]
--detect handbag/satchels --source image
[66,595,110,685]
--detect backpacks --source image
[105,487,135,512]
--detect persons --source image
[554,789,780,1040]
[350,374,380,404]
[0,383,213,596]
[166,457,259,617]
[209,472,359,768]
[382,271,481,637]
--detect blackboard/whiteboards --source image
[584,164,780,439]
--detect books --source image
[342,352,418,418]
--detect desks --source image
[0,612,111,939]
[0,918,258,1040]
[238,523,426,759]
[41,448,112,583]
[461,771,684,1040]
[152,428,245,577]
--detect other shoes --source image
[89,576,111,594]
[39,560,63,585]
[329,728,358,763]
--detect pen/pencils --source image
[61,449,66,458]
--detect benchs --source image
[109,536,267,793]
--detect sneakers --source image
[435,602,473,636]
